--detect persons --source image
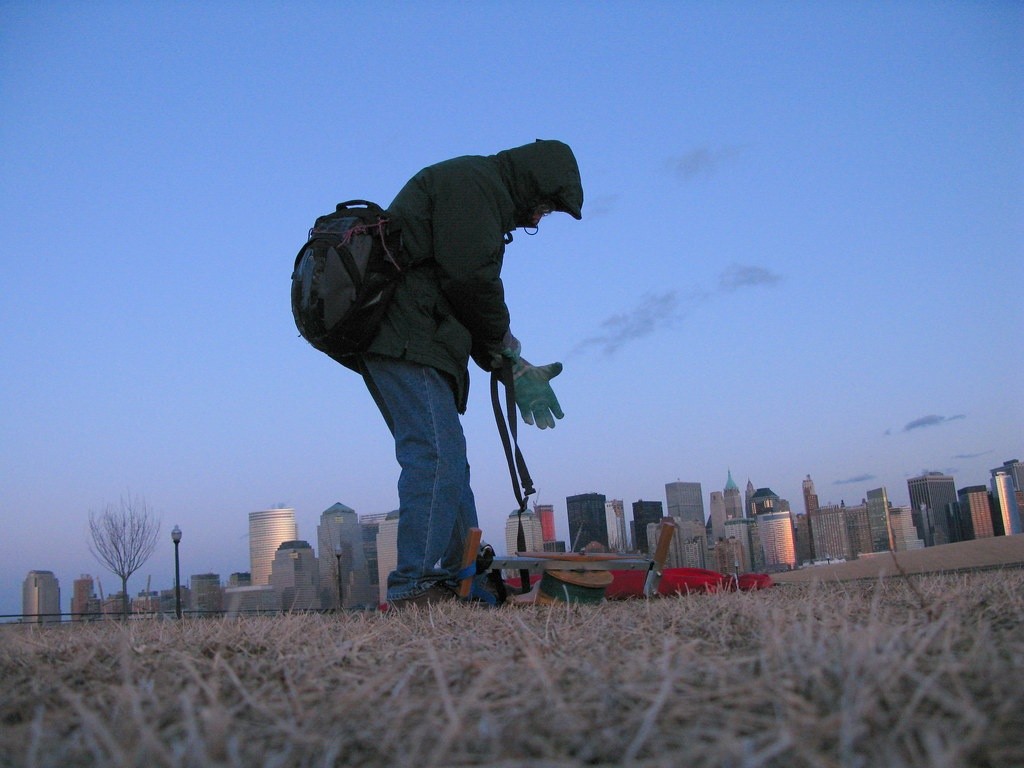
[345,138,585,608]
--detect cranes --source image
[531,488,543,515]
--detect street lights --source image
[170,523,184,620]
[334,543,344,613]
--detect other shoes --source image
[388,586,458,614]
[481,578,532,603]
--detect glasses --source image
[539,201,551,217]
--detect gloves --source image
[509,356,563,430]
[486,330,522,370]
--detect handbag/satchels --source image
[290,197,407,358]
